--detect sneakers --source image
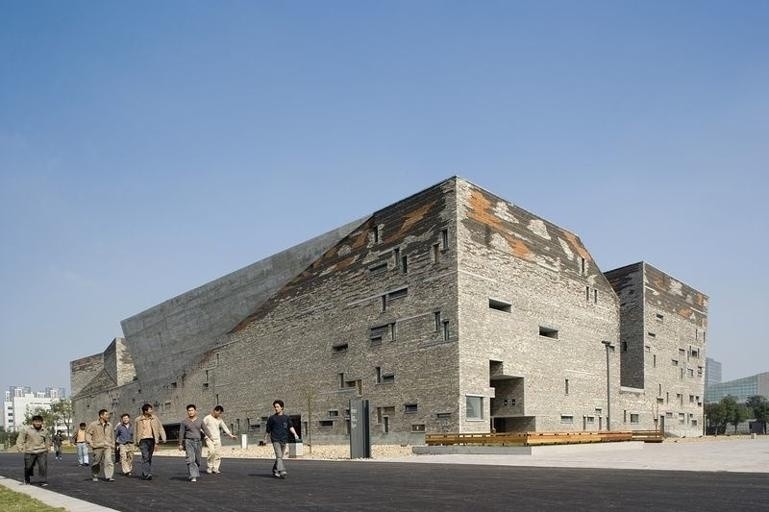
[272,471,288,479]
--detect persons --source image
[202,405,238,475]
[16,414,55,487]
[259,399,302,478]
[132,405,168,479]
[179,404,217,482]
[55,408,135,482]
